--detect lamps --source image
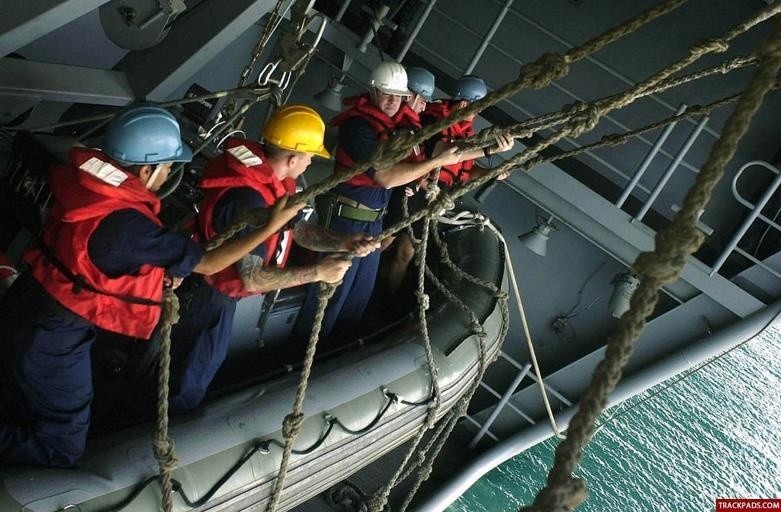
[515,213,560,258]
[313,72,349,113]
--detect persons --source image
[293,62,515,359]
[1,99,351,472]
[138,104,392,422]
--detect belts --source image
[333,196,379,222]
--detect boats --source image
[1,157,514,512]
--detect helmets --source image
[103,102,192,167]
[369,62,435,100]
[453,75,488,101]
[262,105,331,159]
